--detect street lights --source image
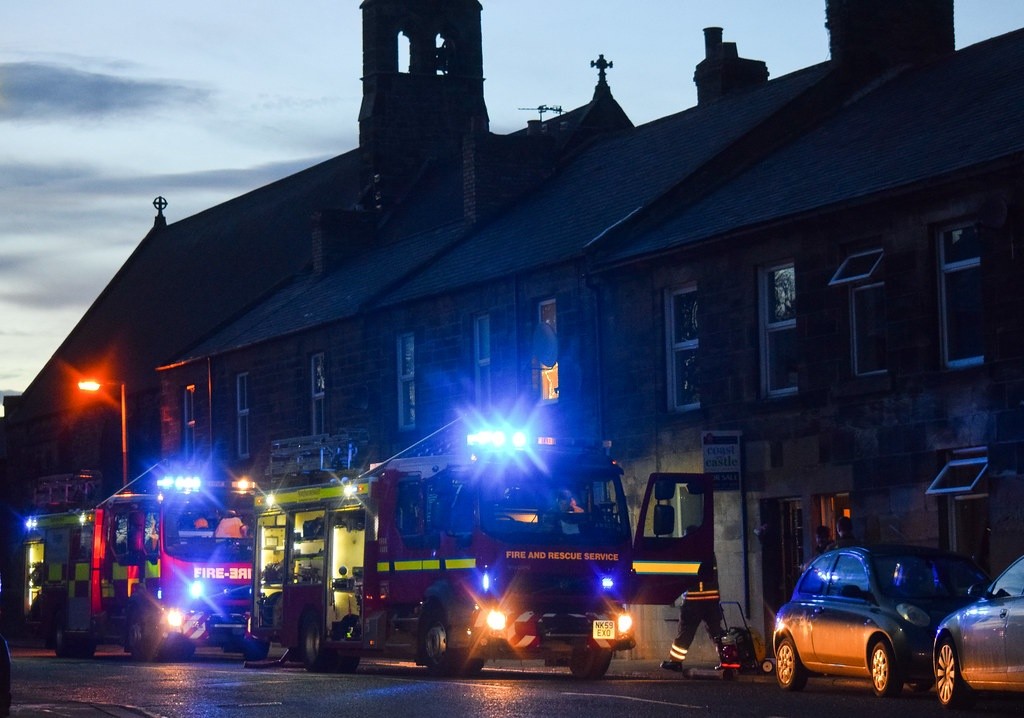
[77,379,128,486]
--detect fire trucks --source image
[24,473,269,660]
[247,428,713,678]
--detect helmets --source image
[685,524,699,536]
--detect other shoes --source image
[660,661,682,671]
[715,664,721,669]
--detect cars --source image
[772,542,993,697]
[932,554,1024,707]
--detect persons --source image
[660,525,728,670]
[813,517,861,553]
